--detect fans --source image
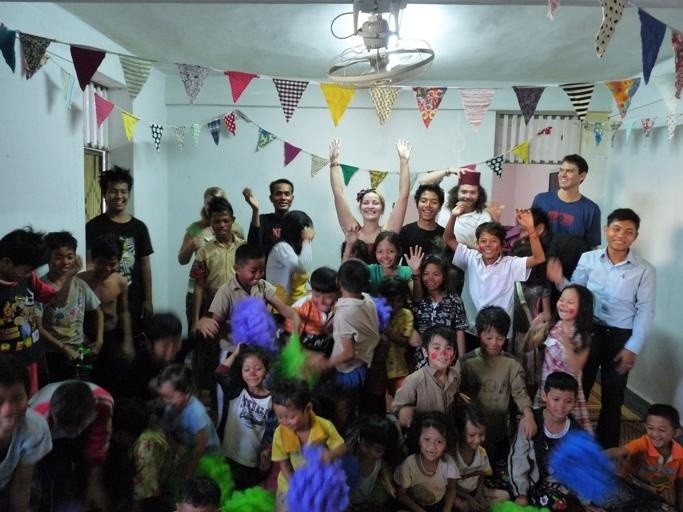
[325,0,436,90]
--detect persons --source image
[2,139,683,510]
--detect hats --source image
[458,171,480,185]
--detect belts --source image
[588,325,632,336]
